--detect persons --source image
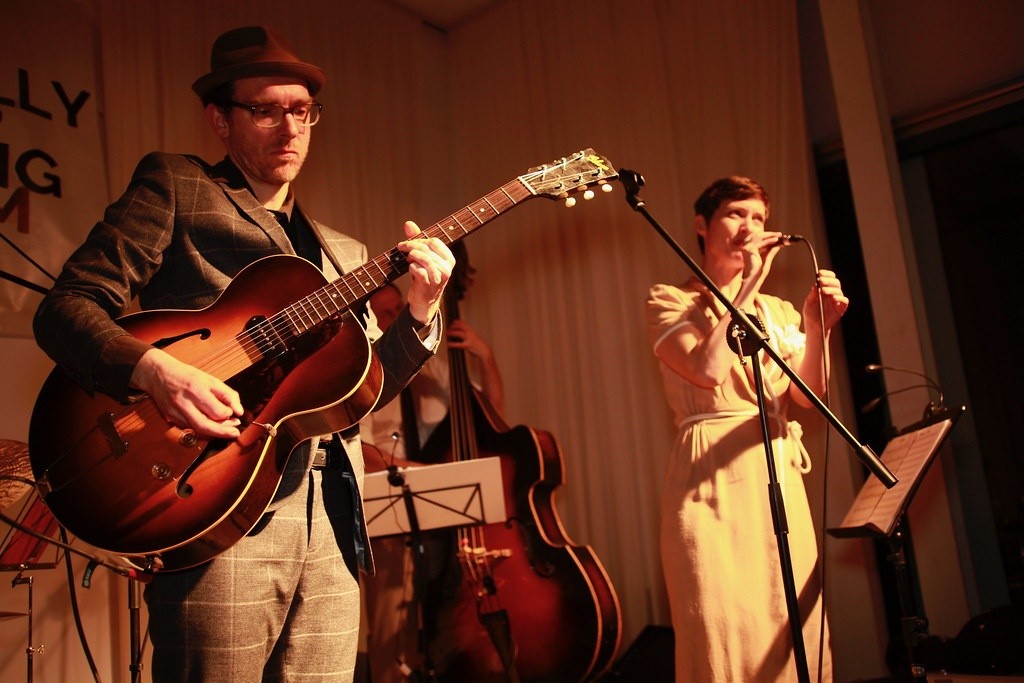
[647,175,849,683]
[355,283,505,683]
[33,26,457,683]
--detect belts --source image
[311,448,352,467]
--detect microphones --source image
[768,235,803,250]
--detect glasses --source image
[233,100,322,129]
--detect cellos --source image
[398,240,625,683]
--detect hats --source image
[191,26,329,100]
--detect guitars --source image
[29,145,619,576]
